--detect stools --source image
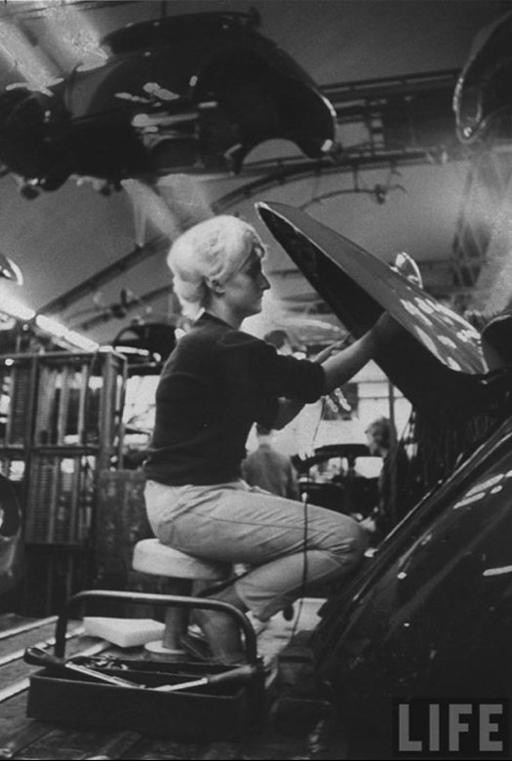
[132,538,230,663]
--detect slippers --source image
[178,633,270,676]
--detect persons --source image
[240,422,301,621]
[143,214,422,667]
[355,416,424,549]
